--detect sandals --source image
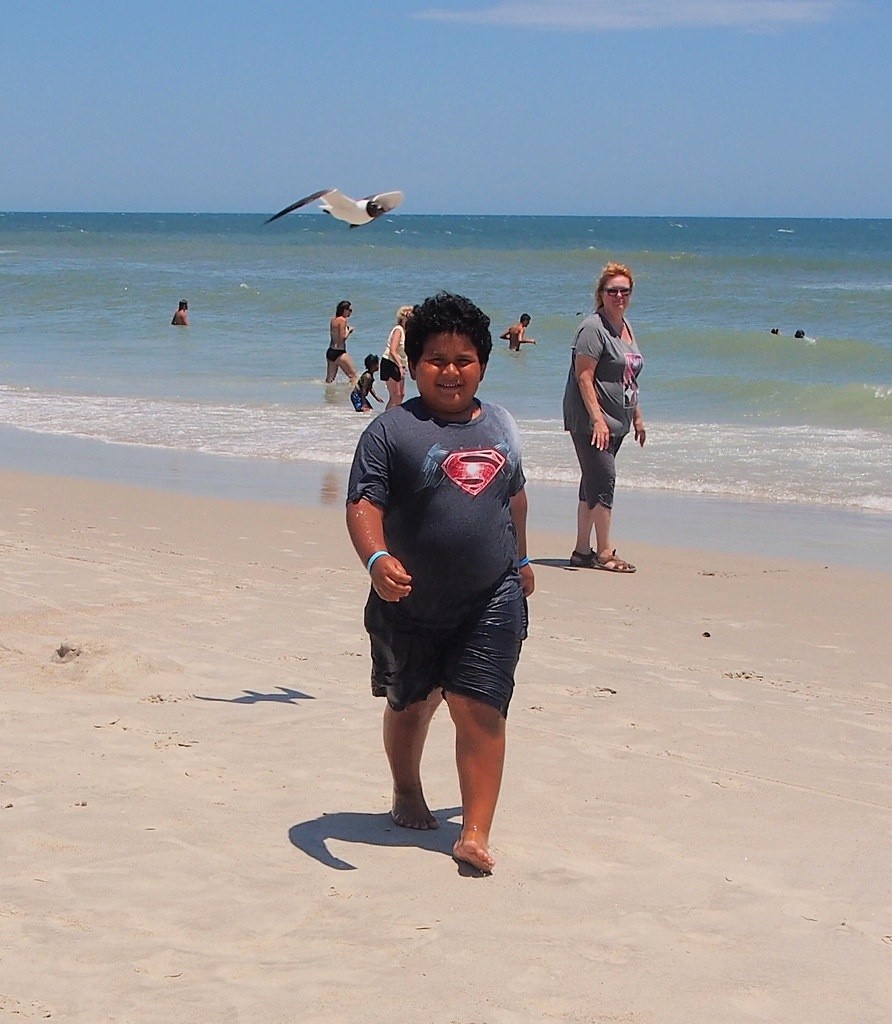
[570,548,637,573]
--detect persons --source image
[380,305,413,412]
[171,300,190,326]
[347,291,536,874]
[563,262,646,573]
[351,353,386,412]
[326,300,359,384]
[500,314,536,352]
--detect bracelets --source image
[519,557,529,567]
[367,551,392,576]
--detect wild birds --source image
[262,188,405,230]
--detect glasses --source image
[347,308,352,312]
[604,288,632,297]
